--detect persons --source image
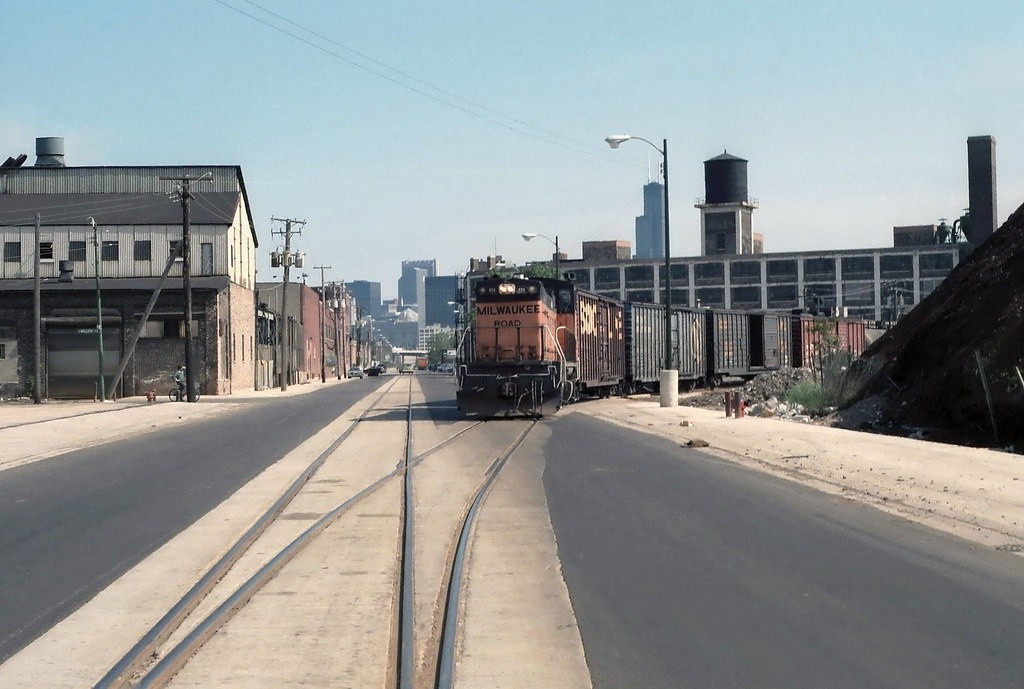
[172,365,187,401]
[397,362,404,374]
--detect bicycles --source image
[169,385,200,402]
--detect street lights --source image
[522,233,559,279]
[605,134,678,407]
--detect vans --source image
[399,363,413,374]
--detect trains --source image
[454,268,866,421]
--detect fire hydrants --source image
[145,391,154,402]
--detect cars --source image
[348,364,387,379]
